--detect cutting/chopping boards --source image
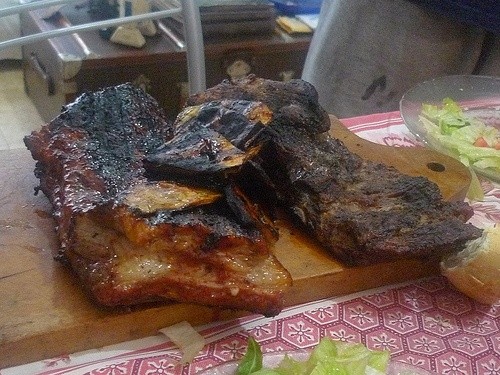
[0,114,472,368]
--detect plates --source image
[399,75,500,183]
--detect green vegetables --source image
[420,99,500,171]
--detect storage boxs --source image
[20,1,315,123]
